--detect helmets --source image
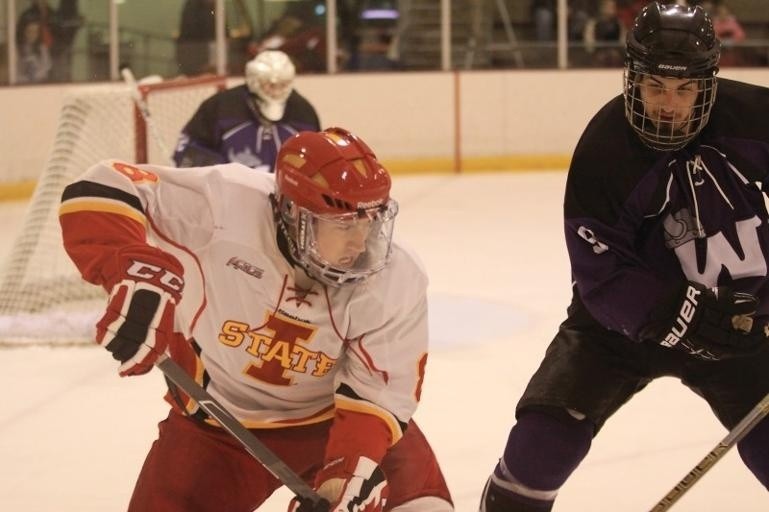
[622,1,722,154]
[244,49,296,121]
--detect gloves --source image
[634,269,767,363]
[92,243,191,378]
[285,455,390,512]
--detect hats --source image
[272,125,400,290]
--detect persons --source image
[173,0,358,79]
[54,126,460,511]
[528,0,749,50]
[17,0,95,88]
[472,2,768,511]
[165,48,326,183]
